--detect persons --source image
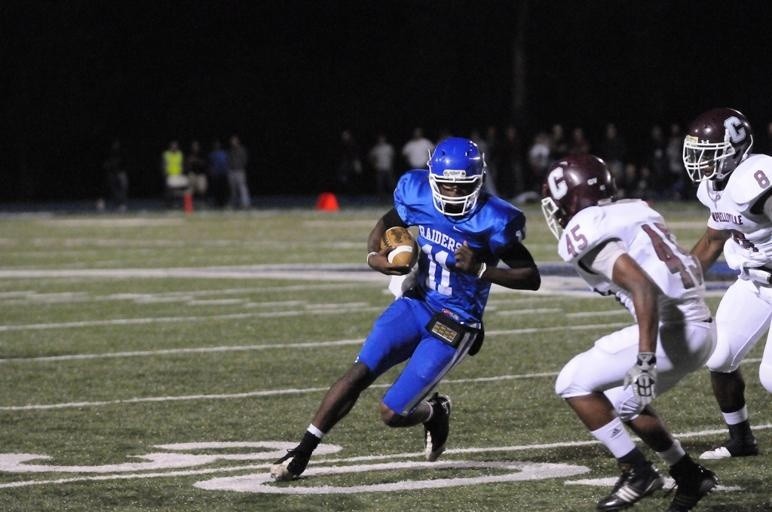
[268,134,542,482]
[92,122,687,215]
[539,151,721,510]
[680,103,772,461]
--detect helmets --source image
[426,136,487,216]
[683,108,754,183]
[540,154,618,241]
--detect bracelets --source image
[478,262,488,279]
[366,251,378,264]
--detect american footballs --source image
[379,226,418,268]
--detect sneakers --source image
[271,450,309,481]
[665,463,718,512]
[423,392,451,461]
[699,437,758,459]
[597,462,663,511]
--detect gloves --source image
[624,352,657,405]
